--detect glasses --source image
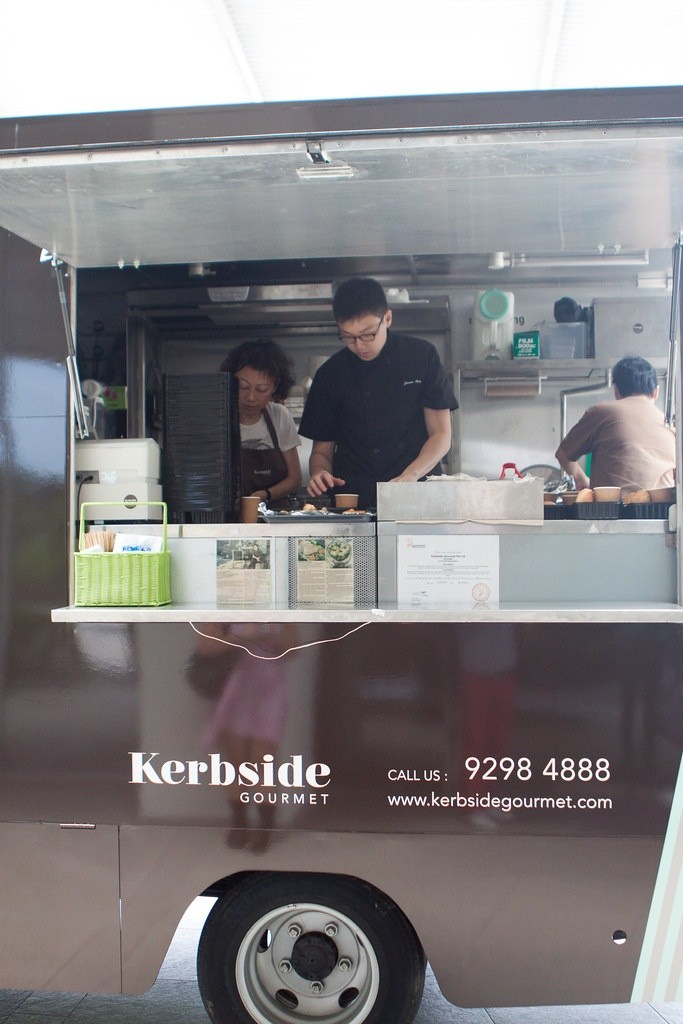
[337,310,388,345]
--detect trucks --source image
[0,82,683,1024]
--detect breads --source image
[623,490,651,504]
[576,488,594,502]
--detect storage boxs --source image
[588,296,671,358]
[163,372,242,524]
[539,321,589,360]
[543,501,675,521]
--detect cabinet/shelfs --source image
[125,294,460,475]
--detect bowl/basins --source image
[326,539,352,567]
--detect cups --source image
[334,494,359,507]
[239,497,260,524]
[593,486,622,503]
[648,486,676,504]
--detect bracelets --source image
[262,488,272,508]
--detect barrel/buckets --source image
[470,286,514,364]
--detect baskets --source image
[74,502,172,606]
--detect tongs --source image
[499,462,524,479]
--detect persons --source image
[221,340,303,523]
[555,356,676,492]
[297,278,459,522]
[247,544,264,569]
